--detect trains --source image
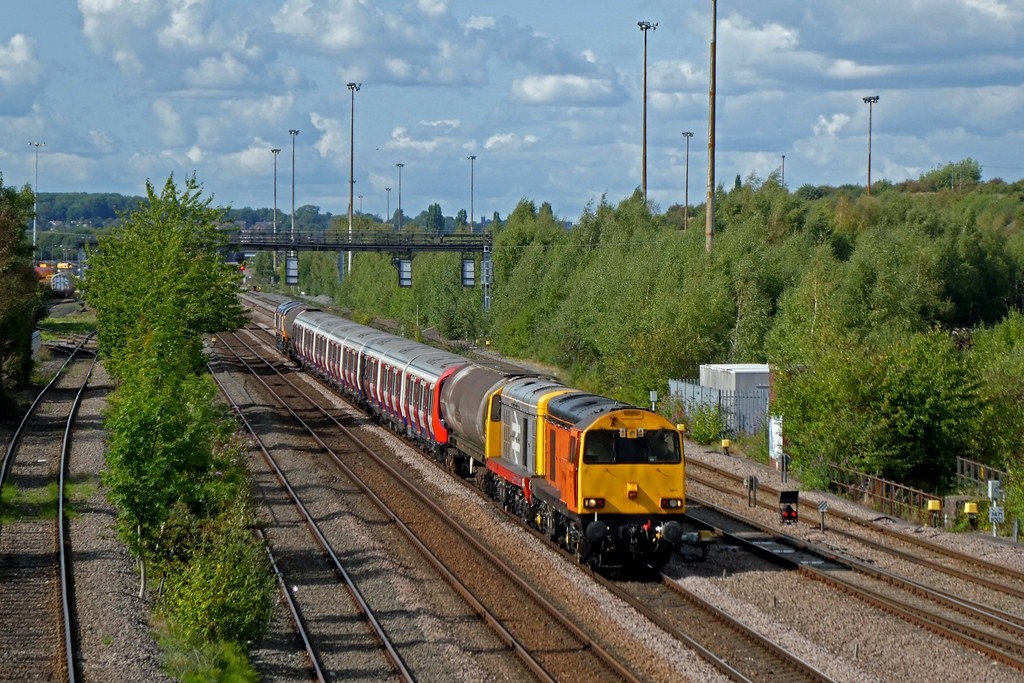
[274,300,688,574]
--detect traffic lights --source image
[784,507,800,522]
[239,267,246,271]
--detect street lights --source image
[289,130,300,242]
[27,141,47,265]
[782,155,786,186]
[359,194,363,216]
[385,188,392,223]
[682,132,695,231]
[348,82,363,245]
[862,96,879,196]
[467,156,477,232]
[637,21,660,204]
[396,163,404,243]
[270,148,281,239]
